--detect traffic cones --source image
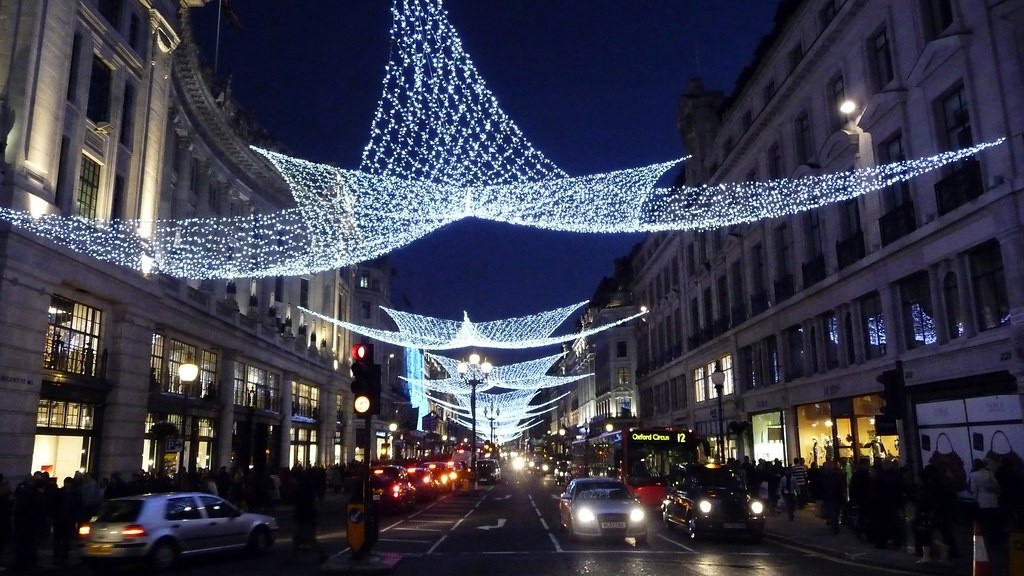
[974,525,993,576]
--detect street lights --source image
[484,402,499,444]
[457,353,493,472]
[712,360,725,464]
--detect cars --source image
[349,444,500,515]
[513,453,571,485]
[661,487,765,543]
[77,492,278,576]
[559,476,648,544]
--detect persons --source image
[0,454,454,576]
[808,434,887,469]
[549,451,1024,576]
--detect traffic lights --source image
[351,344,375,419]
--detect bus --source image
[570,428,711,507]
